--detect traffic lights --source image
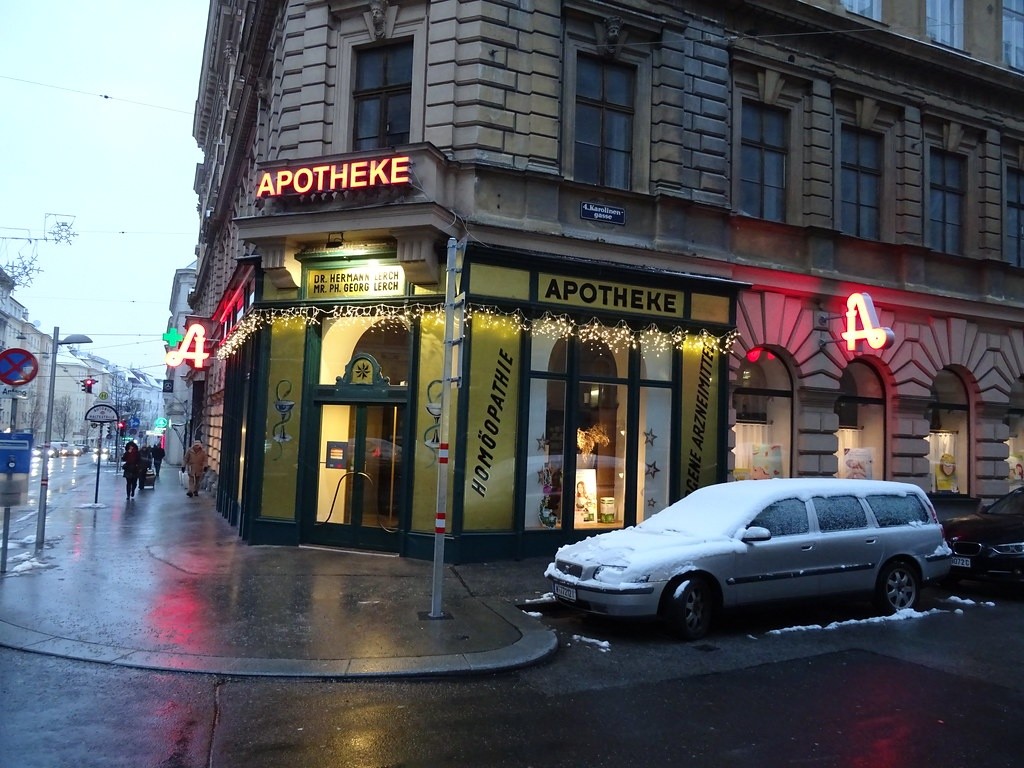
[119,422,126,437]
[84,378,93,394]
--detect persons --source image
[181,440,208,497]
[576,482,591,512]
[122,442,165,500]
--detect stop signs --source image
[1,348,38,387]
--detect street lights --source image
[34,326,93,545]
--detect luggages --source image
[145,468,155,489]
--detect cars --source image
[543,474,953,642]
[939,487,1024,600]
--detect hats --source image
[157,442,161,446]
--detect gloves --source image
[204,467,207,471]
[182,467,185,472]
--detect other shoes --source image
[126,493,134,499]
[186,491,198,497]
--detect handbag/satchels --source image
[179,471,189,489]
